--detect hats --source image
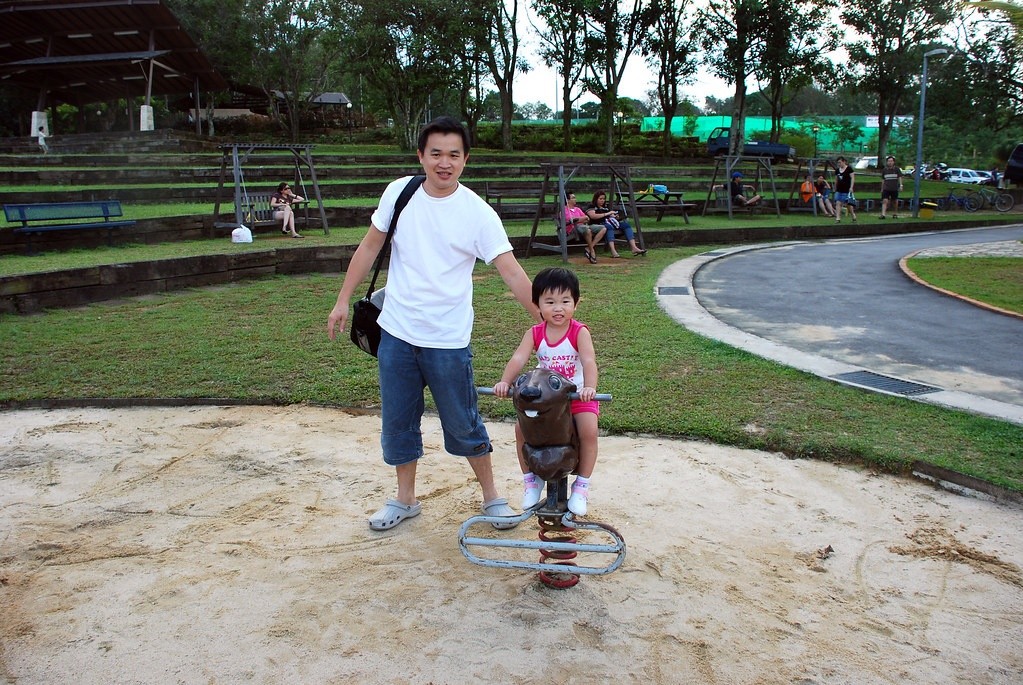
[732,172,743,178]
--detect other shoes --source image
[521,475,545,511]
[292,235,304,238]
[282,231,287,234]
[834,220,841,224]
[568,481,589,517]
[879,214,885,219]
[851,218,856,222]
[893,215,897,218]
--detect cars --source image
[854,156,1004,185]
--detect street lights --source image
[347,103,353,142]
[913,49,947,218]
[617,112,624,148]
[812,125,819,157]
[864,145,868,155]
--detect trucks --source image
[707,127,797,165]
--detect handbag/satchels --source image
[350,286,387,358]
[232,224,252,243]
[845,191,857,207]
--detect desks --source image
[614,191,689,225]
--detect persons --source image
[800,175,837,217]
[328,119,542,530]
[38,126,53,154]
[583,190,647,258]
[559,191,607,264]
[269,182,306,238]
[878,155,903,219]
[833,156,857,223]
[713,171,761,206]
[991,167,999,187]
[493,266,599,516]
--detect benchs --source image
[610,201,696,211]
[485,179,560,224]
[3,199,136,246]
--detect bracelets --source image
[294,195,297,199]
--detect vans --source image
[1004,142,1023,181]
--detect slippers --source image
[585,248,595,260]
[586,252,597,263]
[368,498,421,530]
[481,498,518,529]
[632,249,647,257]
[610,254,621,258]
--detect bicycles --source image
[938,182,1015,212]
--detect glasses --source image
[284,187,291,190]
